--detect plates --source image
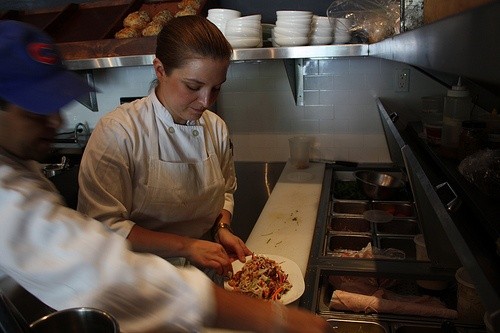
[224,254,305,305]
[205,8,263,48]
[272,10,353,47]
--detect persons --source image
[0,19,336,332]
[74,14,253,292]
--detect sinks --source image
[54,139,86,155]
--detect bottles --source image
[442,76,470,140]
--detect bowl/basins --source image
[421,96,444,138]
[353,171,406,200]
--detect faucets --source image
[75,123,86,142]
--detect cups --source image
[288,137,311,169]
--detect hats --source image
[0,20,104,117]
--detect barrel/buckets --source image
[414,234,429,260]
[29,308,120,333]
[455,266,484,321]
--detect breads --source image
[114,0,202,38]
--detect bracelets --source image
[270,303,290,332]
[213,221,235,237]
